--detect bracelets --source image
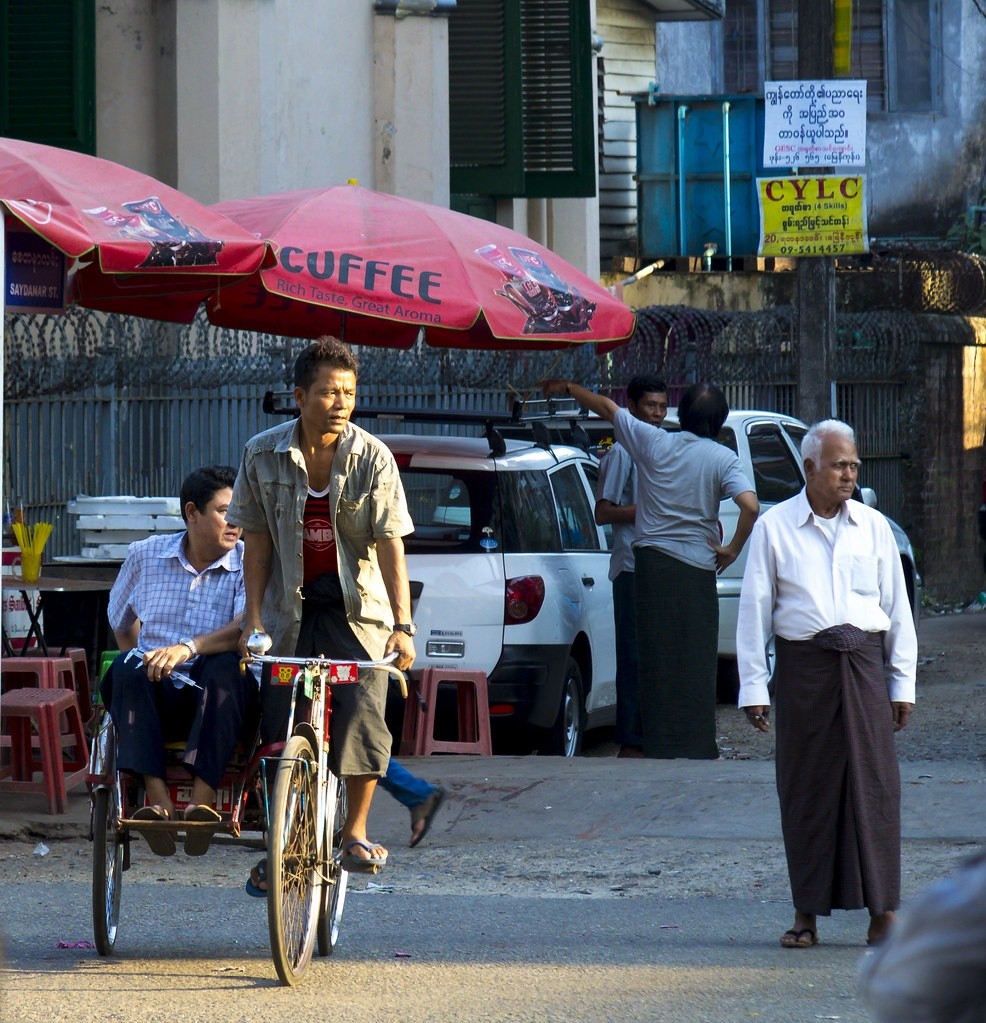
[746,710,770,720]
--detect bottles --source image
[12,495,24,546]
[2,495,13,547]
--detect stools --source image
[0,646,92,816]
[400,666,491,754]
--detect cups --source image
[11,554,42,585]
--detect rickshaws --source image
[80,627,411,988]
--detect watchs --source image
[393,622,418,637]
[179,637,198,655]
[564,380,575,395]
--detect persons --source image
[232,336,447,899]
[734,417,920,949]
[100,465,268,857]
[534,374,760,761]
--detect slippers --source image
[343,841,389,865]
[411,782,446,848]
[780,929,820,947]
[131,805,176,857]
[246,857,271,897]
[183,805,221,857]
[867,934,884,949]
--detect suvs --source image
[258,390,623,760]
[536,410,922,703]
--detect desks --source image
[2,575,118,658]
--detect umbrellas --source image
[0,134,279,276]
[70,181,637,355]
